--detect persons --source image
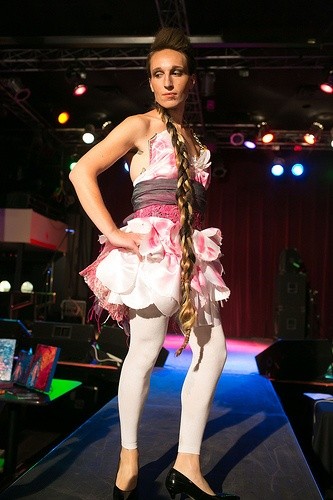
[69,28,231,500]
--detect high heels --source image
[112,469,139,500]
[165,468,240,500]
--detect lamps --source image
[0,79,30,102]
[65,66,88,97]
[81,125,95,144]
[304,122,324,145]
[231,122,274,149]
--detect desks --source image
[0,378,83,485]
